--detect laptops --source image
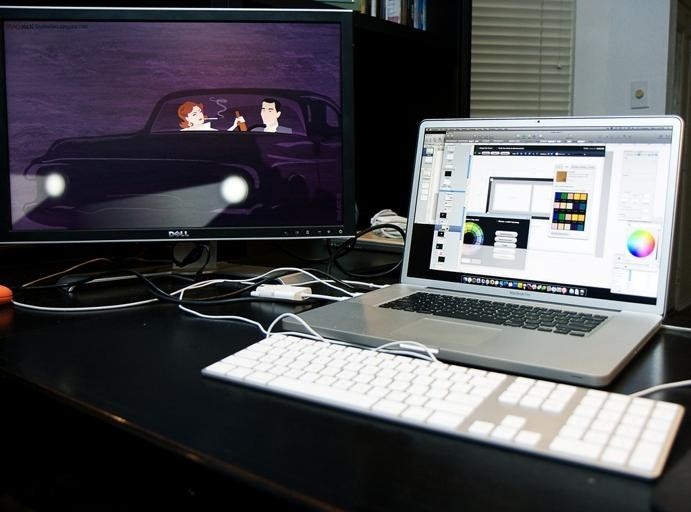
[281,114,685,388]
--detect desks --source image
[0,251,690,511]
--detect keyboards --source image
[201,334,687,481]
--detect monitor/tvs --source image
[0,6,358,292]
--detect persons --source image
[250,98,292,134]
[178,102,246,131]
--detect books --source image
[373,1,427,31]
